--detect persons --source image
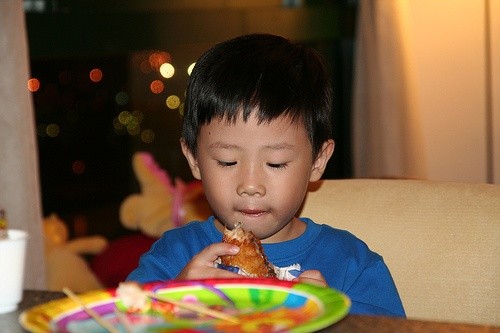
[124,33,406,320]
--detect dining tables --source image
[0,288,500,333]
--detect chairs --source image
[292,175,500,327]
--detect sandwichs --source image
[220,226,278,280]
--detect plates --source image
[19,277,351,333]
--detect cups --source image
[0,229,27,313]
